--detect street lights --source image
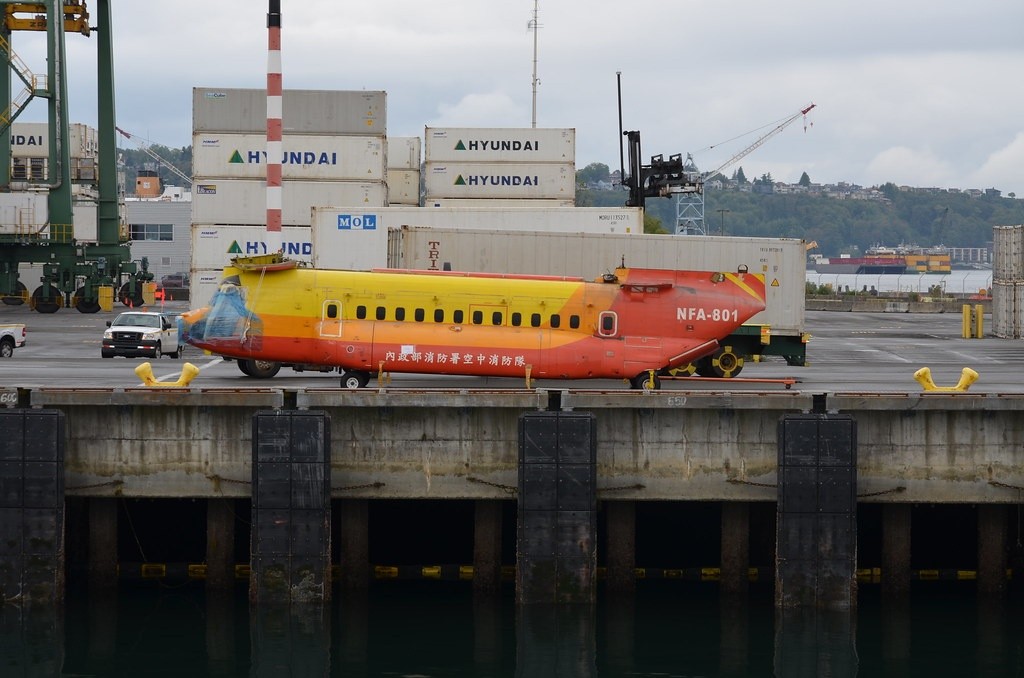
[835,274,839,295]
[855,274,858,291]
[919,274,924,293]
[940,274,946,298]
[818,274,822,287]
[878,274,882,291]
[897,274,902,291]
[963,272,970,299]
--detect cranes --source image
[675,102,817,236]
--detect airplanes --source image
[175,252,766,390]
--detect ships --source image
[816,245,951,275]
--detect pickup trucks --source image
[0,323,26,357]
[101,312,185,359]
